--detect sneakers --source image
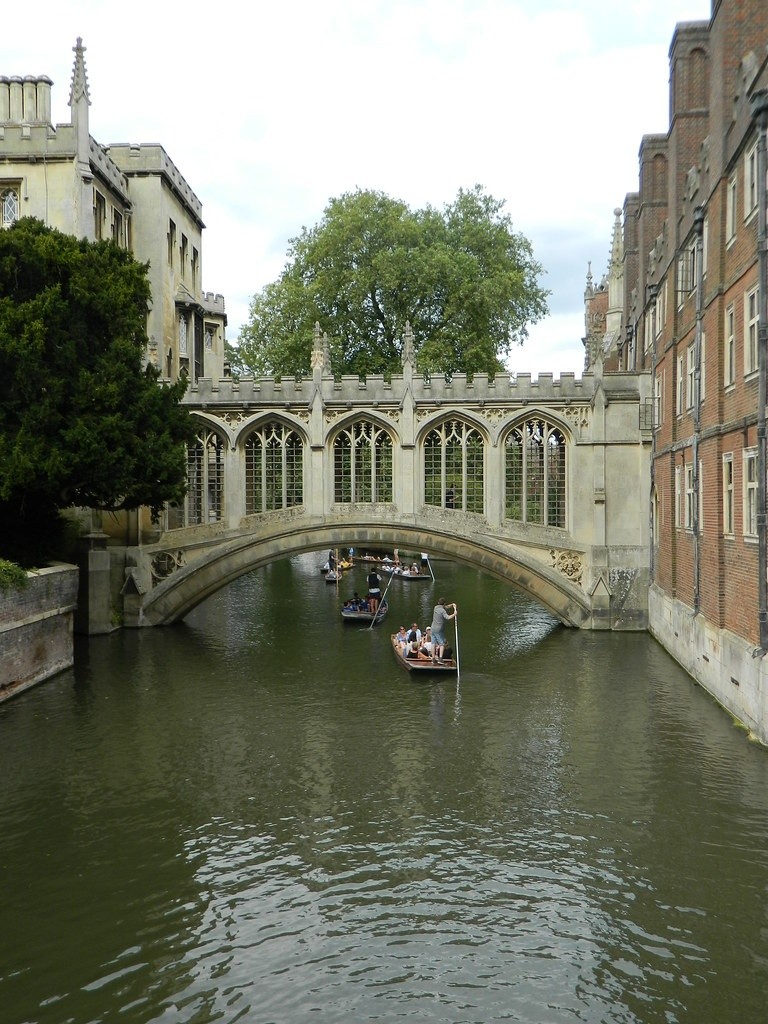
[437,658,448,665]
[431,658,438,664]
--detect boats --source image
[375,564,432,579]
[340,598,389,625]
[352,556,402,566]
[321,561,354,571]
[325,570,344,581]
[390,632,459,672]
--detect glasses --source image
[400,629,404,630]
[413,626,418,627]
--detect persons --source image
[446,484,459,508]
[344,592,386,612]
[328,548,353,579]
[382,563,420,575]
[361,553,390,561]
[396,623,453,659]
[431,598,457,664]
[394,549,399,562]
[367,568,382,615]
[420,552,428,576]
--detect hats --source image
[426,627,431,630]
